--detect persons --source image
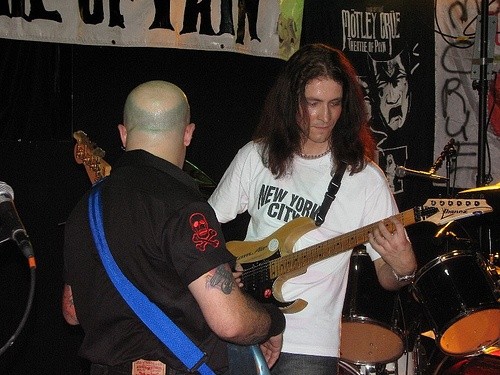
[208,44,419,375]
[62,81,285,375]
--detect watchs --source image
[392,264,417,282]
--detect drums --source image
[341,248,407,365]
[410,249,500,355]
[428,342,500,375]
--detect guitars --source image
[73,130,111,186]
[226,197,493,314]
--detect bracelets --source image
[263,303,285,335]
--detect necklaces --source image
[297,148,332,158]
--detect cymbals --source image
[458,182,500,195]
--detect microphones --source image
[433,220,455,240]
[395,166,448,183]
[0,182,36,267]
[428,138,455,173]
